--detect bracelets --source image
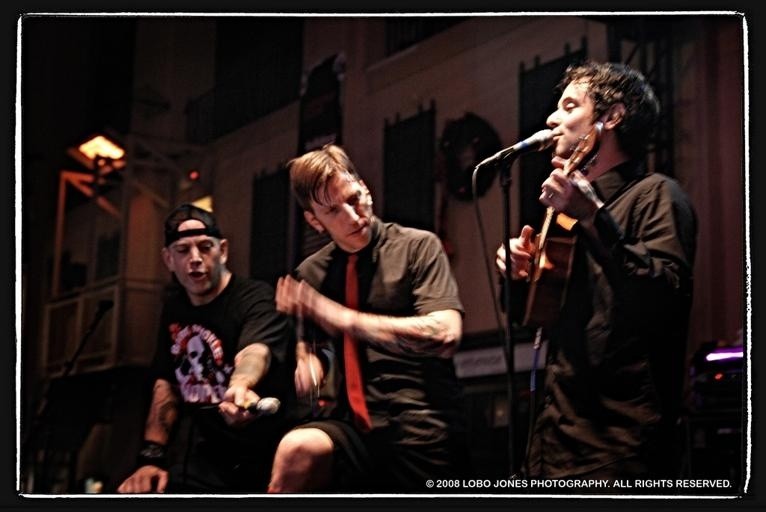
[139,442,165,470]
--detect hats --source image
[164,203,221,247]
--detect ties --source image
[343,255,373,430]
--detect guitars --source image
[522,123,603,329]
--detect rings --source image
[548,192,555,199]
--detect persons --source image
[267,143,466,496]
[494,62,698,494]
[118,203,296,493]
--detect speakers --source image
[686,409,743,496]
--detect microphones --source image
[200,396,281,416]
[474,129,554,171]
[85,299,114,339]
